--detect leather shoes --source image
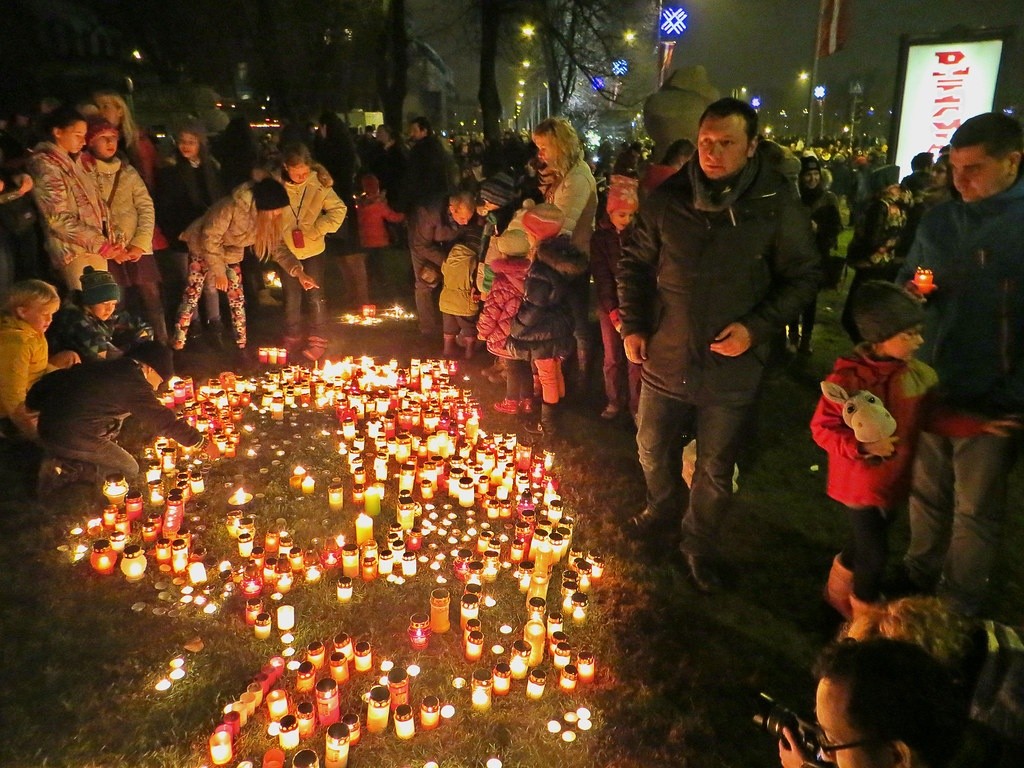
[681,535,730,592]
[617,509,679,540]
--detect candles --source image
[52,345,615,767]
[910,272,936,295]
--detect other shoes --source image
[790,341,814,353]
[599,405,618,419]
[281,336,304,353]
[236,348,260,370]
[482,362,507,383]
[302,336,328,360]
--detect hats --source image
[497,229,530,255]
[481,172,515,207]
[177,117,206,145]
[359,174,378,194]
[253,178,290,210]
[607,183,639,212]
[88,115,119,146]
[508,199,535,230]
[523,204,565,240]
[125,340,174,382]
[856,279,930,342]
[800,157,821,177]
[80,266,120,305]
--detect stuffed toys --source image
[819,382,901,462]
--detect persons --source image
[268,139,347,361]
[599,138,697,191]
[810,278,1018,624]
[0,76,531,493]
[894,112,1024,617]
[474,229,539,414]
[591,173,651,430]
[779,593,1024,768]
[616,99,825,595]
[532,116,598,399]
[26,106,129,294]
[800,142,959,273]
[523,201,590,435]
[354,175,406,308]
[166,176,320,369]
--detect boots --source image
[523,402,562,435]
[439,334,455,358]
[463,337,476,359]
[189,319,227,351]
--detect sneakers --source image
[494,399,533,414]
